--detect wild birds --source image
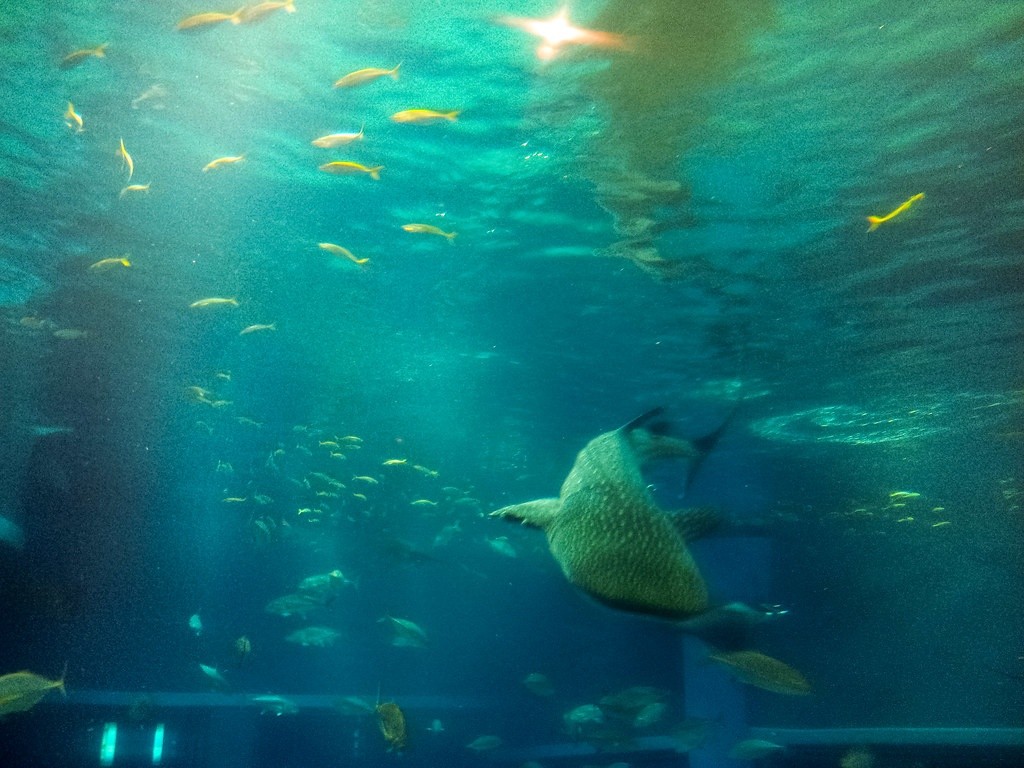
[2,0,928,765]
